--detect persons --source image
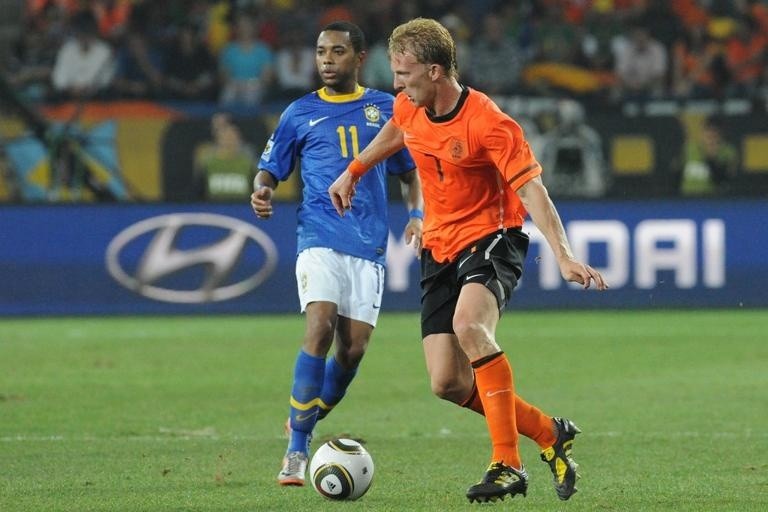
[328,18,609,503]
[0,0,768,101]
[250,21,423,485]
[678,121,737,195]
[534,97,608,198]
[195,110,260,198]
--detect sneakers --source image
[286,417,313,449]
[466,459,529,504]
[541,416,581,501]
[277,450,309,486]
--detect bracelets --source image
[409,208,423,220]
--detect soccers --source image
[310,439,375,500]
